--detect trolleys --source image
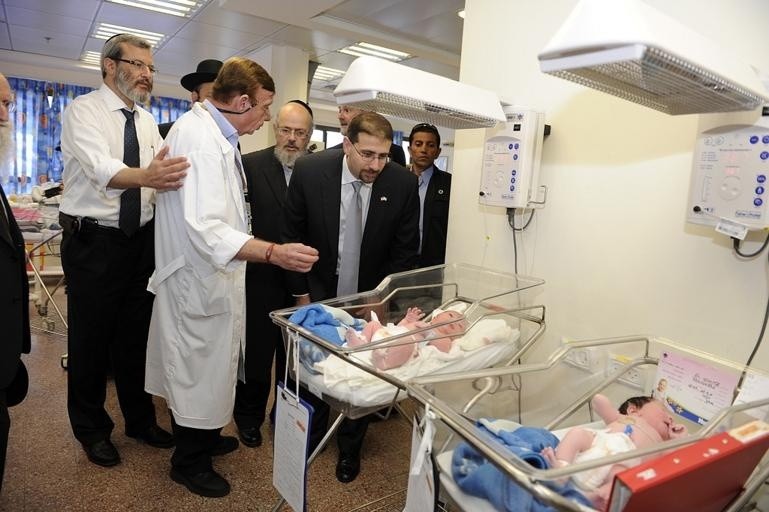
[25,229,68,370]
[267,261,547,511]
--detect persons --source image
[0,74,31,492]
[158,59,224,140]
[345,307,468,370]
[60,33,191,467]
[241,100,452,482]
[540,394,690,511]
[654,380,667,401]
[144,56,319,499]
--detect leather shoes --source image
[82,439,121,467]
[238,426,261,447]
[170,466,230,497]
[336,457,360,483]
[124,425,176,449]
[210,436,239,456]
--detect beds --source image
[21,230,67,278]
[271,261,547,419]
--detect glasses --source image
[275,126,311,139]
[349,137,392,164]
[0,100,17,113]
[255,102,273,121]
[110,57,158,76]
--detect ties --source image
[337,181,362,298]
[119,107,141,239]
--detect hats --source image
[181,59,223,92]
